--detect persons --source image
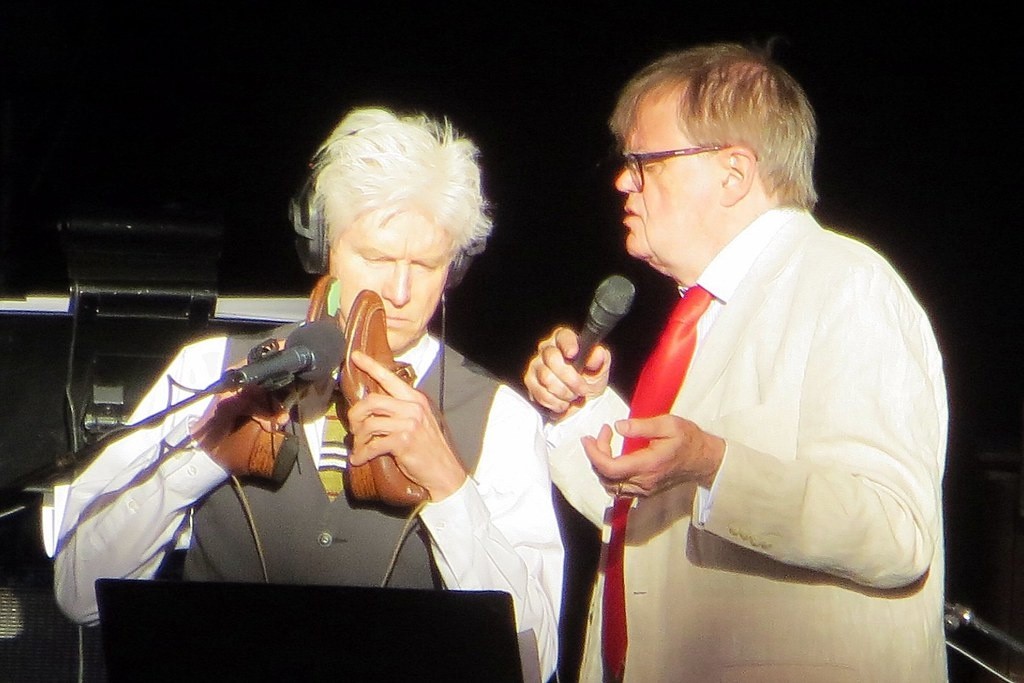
[524,45,948,683]
[56,108,565,683]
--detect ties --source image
[601,284,714,683]
[317,397,350,502]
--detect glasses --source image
[625,144,760,192]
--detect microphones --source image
[541,275,635,420]
[230,320,347,390]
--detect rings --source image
[617,482,623,496]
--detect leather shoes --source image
[213,275,346,490]
[338,291,452,509]
